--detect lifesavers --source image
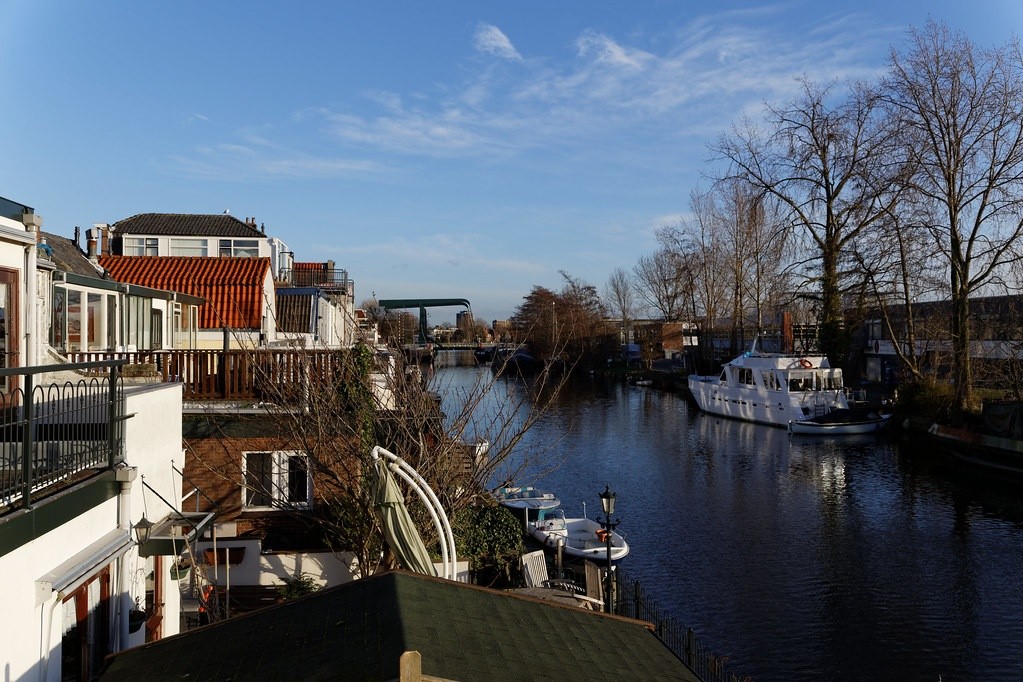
[800,359,813,369]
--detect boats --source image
[686,338,851,428]
[491,484,562,522]
[927,395,1023,486]
[474,348,493,363]
[492,348,534,373]
[524,502,631,571]
[787,405,895,435]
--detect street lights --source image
[598,485,617,616]
